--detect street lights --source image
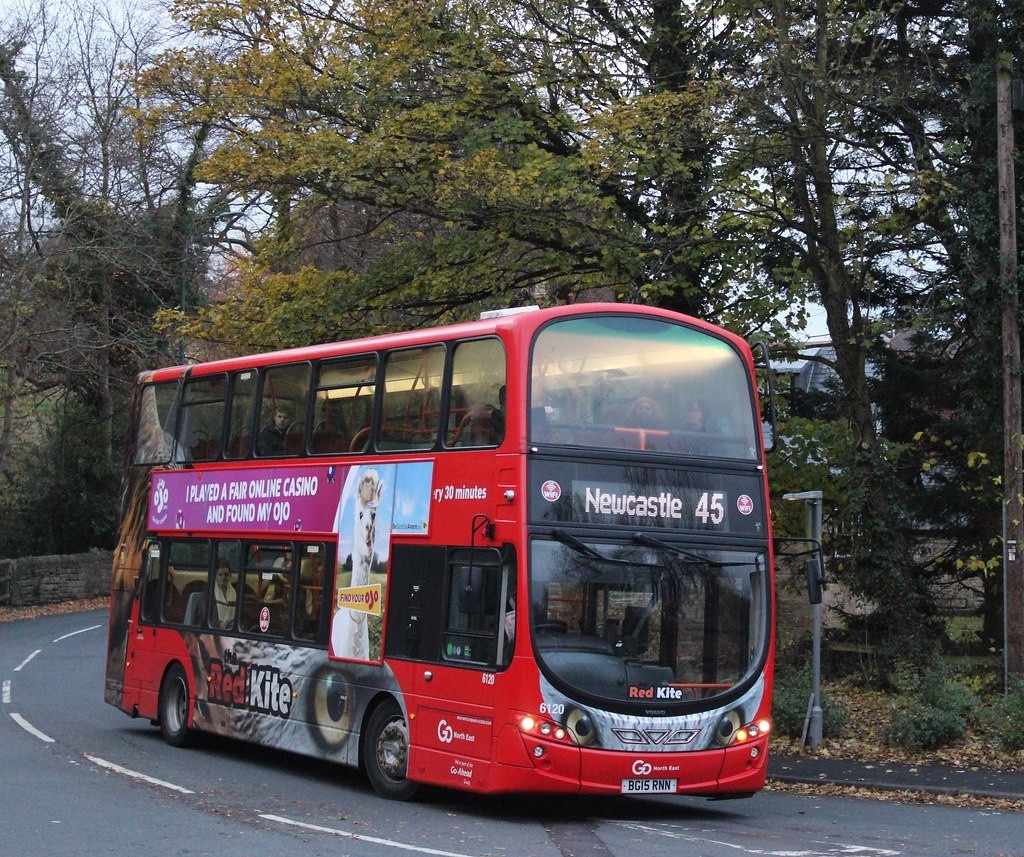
[179,212,244,363]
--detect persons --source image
[258,406,297,456]
[621,397,665,447]
[213,549,313,632]
[670,399,724,452]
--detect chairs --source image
[185,420,351,460]
[145,578,259,633]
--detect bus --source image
[103,302,827,801]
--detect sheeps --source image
[331,468,386,662]
